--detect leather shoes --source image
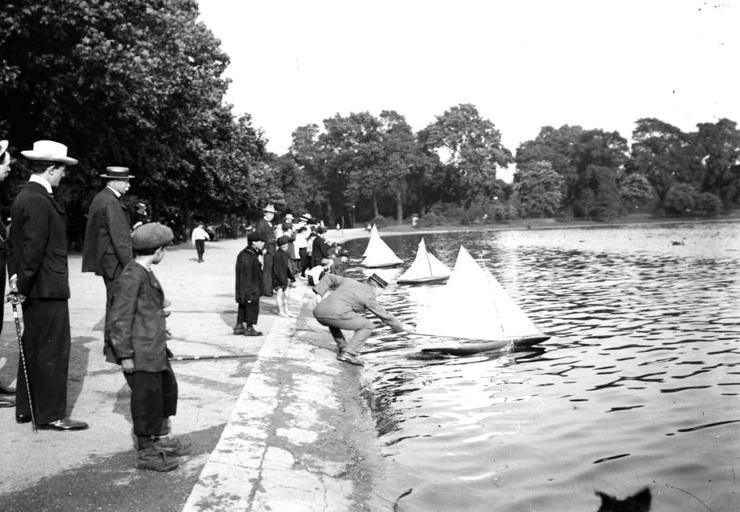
[336,352,364,365]
[0,385,89,431]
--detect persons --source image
[82,166,134,364]
[232,204,367,337]
[103,222,192,472]
[191,221,211,263]
[1,138,18,408]
[6,139,89,431]
[312,272,417,366]
[129,203,150,232]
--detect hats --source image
[369,273,388,289]
[100,166,135,179]
[132,221,174,249]
[20,140,79,165]
[247,204,311,247]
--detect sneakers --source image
[233,327,244,335]
[244,327,262,336]
[156,438,183,456]
[137,448,178,472]
